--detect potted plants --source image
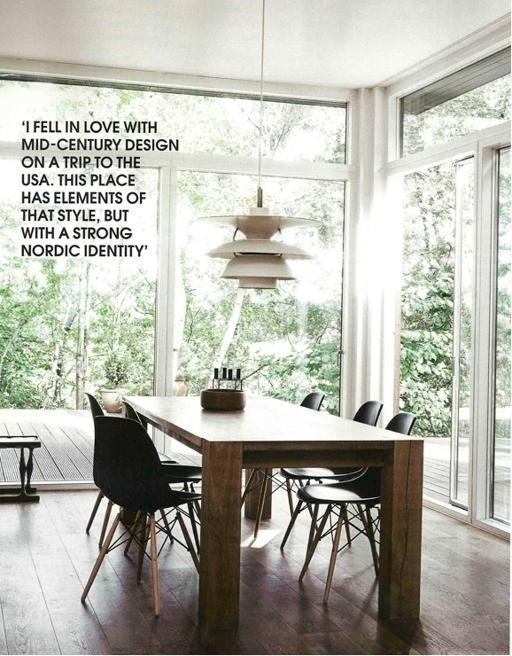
[98,352,131,413]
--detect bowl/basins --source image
[200,388,246,411]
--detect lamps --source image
[192,0,324,292]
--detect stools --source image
[0,435,42,504]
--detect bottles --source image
[212,367,243,391]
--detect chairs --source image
[81,390,203,619]
[242,392,419,604]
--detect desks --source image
[121,393,424,630]
